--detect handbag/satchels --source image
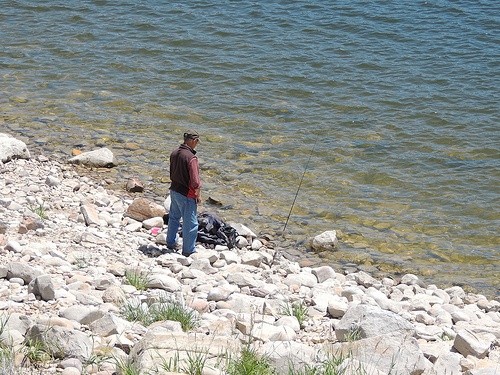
[179,213,240,249]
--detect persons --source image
[165,128,204,257]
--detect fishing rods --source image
[272,132,320,262]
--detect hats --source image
[184,130,203,145]
